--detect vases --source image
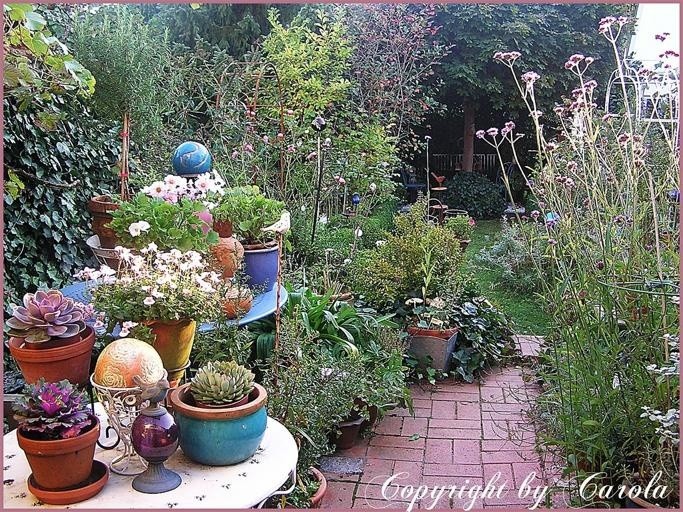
[455,238,472,251]
[165,382,271,466]
[150,320,197,368]
[408,327,457,338]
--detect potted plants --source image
[255,250,414,512]
[66,0,197,249]
[7,289,95,388]
[190,360,255,409]
[12,376,100,489]
[215,186,286,293]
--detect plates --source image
[86,234,135,259]
[22,459,109,506]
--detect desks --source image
[0,401,298,512]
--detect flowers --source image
[139,169,226,211]
[405,296,449,330]
[87,221,222,344]
[445,215,476,239]
